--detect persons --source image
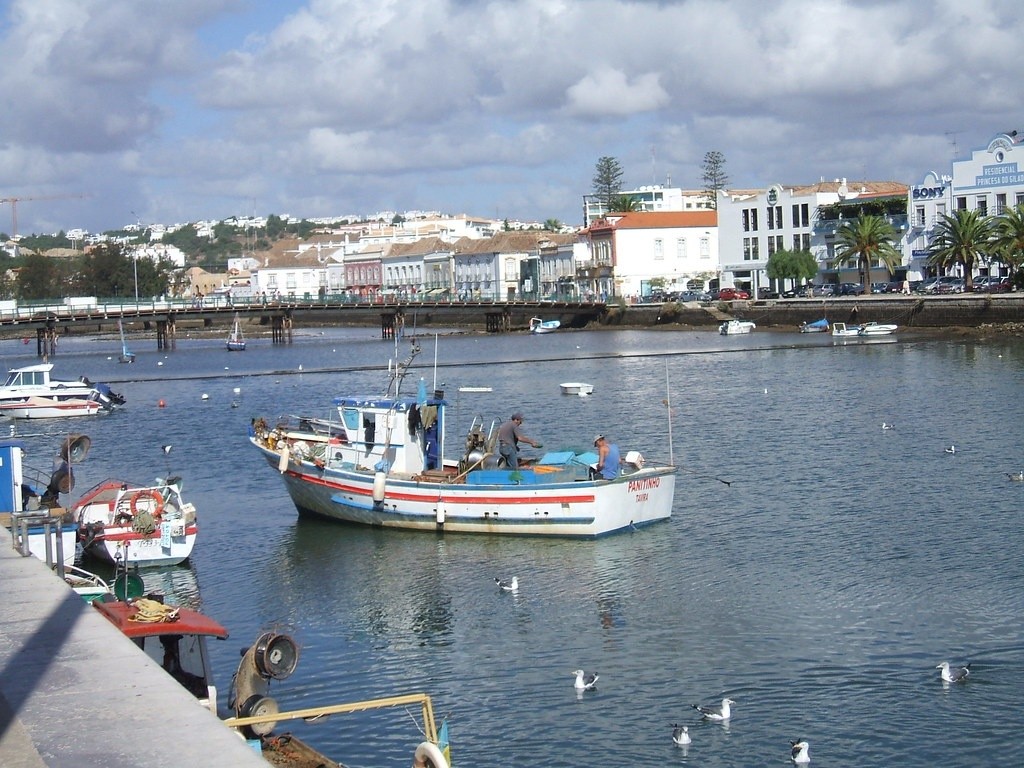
[474,288,480,298]
[593,434,622,480]
[808,281,814,298]
[225,292,231,307]
[902,279,910,295]
[498,412,538,466]
[255,290,281,305]
[601,290,607,303]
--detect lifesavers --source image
[130,490,164,517]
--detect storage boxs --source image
[540,451,576,466]
[572,450,600,466]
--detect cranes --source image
[0,194,79,236]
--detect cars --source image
[636,286,754,304]
[945,278,966,294]
[972,276,1017,294]
[756,280,924,299]
[915,276,948,295]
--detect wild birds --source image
[688,698,735,719]
[936,661,972,683]
[880,422,895,430]
[571,669,599,689]
[1005,471,1023,481]
[788,737,810,763]
[944,445,954,454]
[493,576,520,590]
[669,723,691,744]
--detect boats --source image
[1,417,453,768]
[528,315,561,335]
[831,320,898,336]
[0,350,128,420]
[458,387,494,393]
[718,319,757,335]
[798,318,830,334]
[68,442,199,573]
[247,310,678,543]
[559,383,595,398]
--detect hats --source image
[594,434,604,447]
[511,414,523,419]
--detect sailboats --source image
[117,318,136,364]
[224,311,247,352]
